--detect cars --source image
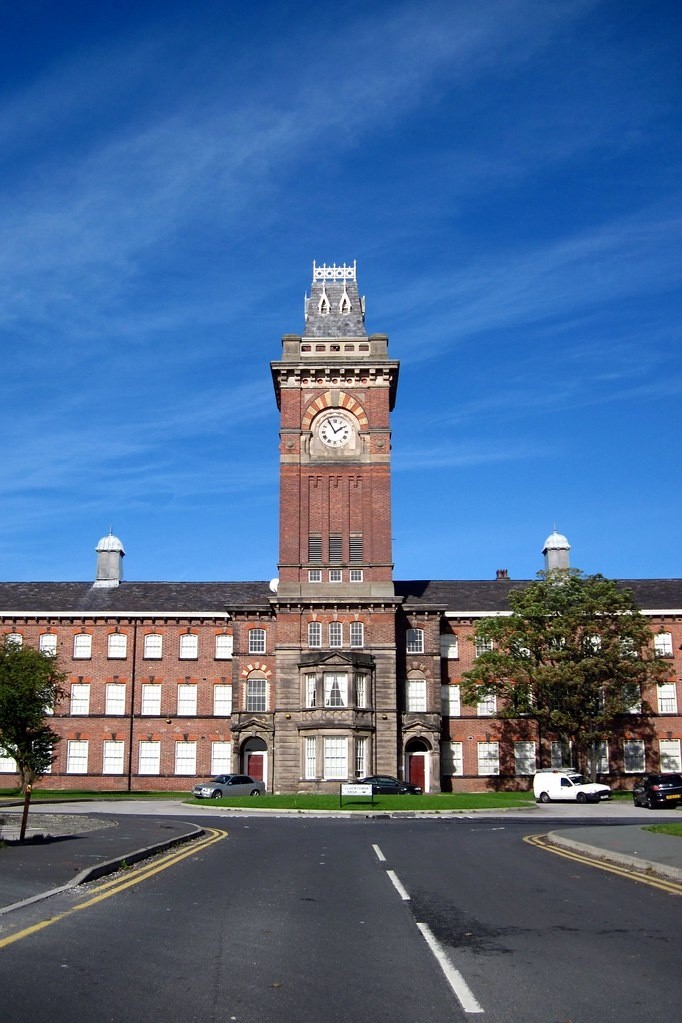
[193,772,266,799]
[358,774,422,795]
[631,773,682,809]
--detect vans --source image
[533,767,612,803]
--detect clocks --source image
[318,414,351,448]
